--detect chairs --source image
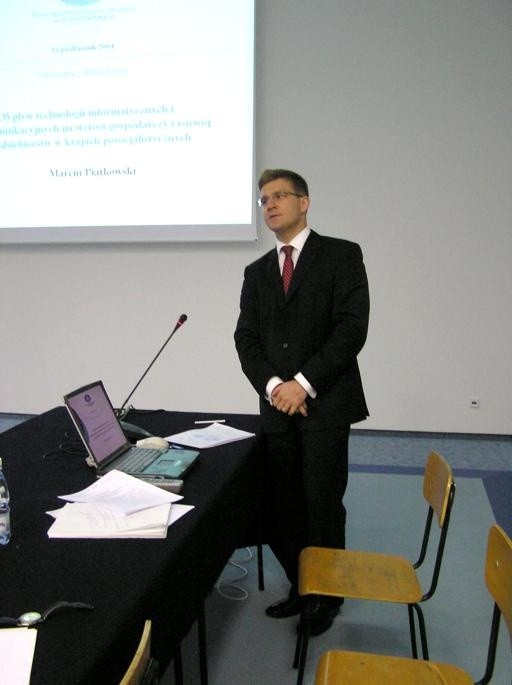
[292,452,456,684]
[316,524,512,685]
[117,619,157,685]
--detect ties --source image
[282,246,294,295]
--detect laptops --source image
[61,380,201,480]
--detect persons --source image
[234,168,370,638]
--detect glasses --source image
[257,190,305,206]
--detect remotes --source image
[144,478,184,488]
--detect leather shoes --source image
[296,598,339,637]
[267,595,300,618]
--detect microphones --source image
[110,312,189,438]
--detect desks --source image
[0,407,269,685]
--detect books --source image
[44,468,195,539]
[162,422,255,450]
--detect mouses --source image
[135,435,170,452]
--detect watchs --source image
[1,601,96,628]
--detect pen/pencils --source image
[194,419,226,424]
